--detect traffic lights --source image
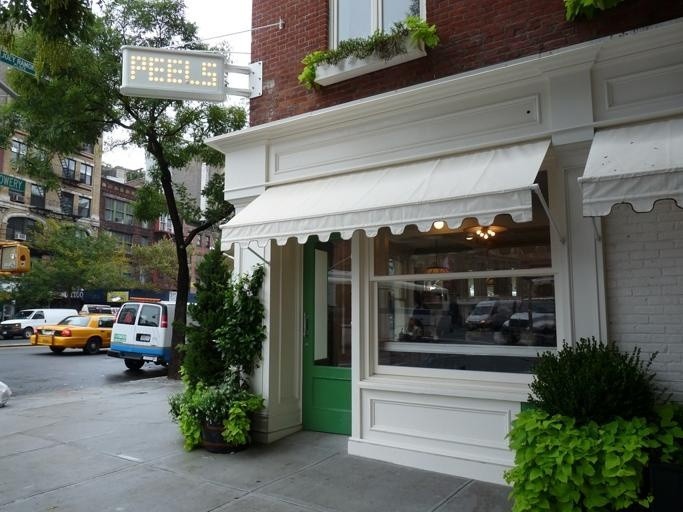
[0,244,30,276]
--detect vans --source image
[0,309,79,340]
[110,297,198,369]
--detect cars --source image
[465,296,555,345]
[31,314,115,354]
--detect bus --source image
[376,281,454,343]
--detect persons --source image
[398,302,552,371]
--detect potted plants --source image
[169,238,265,452]
[298,13,442,90]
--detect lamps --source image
[463,219,508,240]
